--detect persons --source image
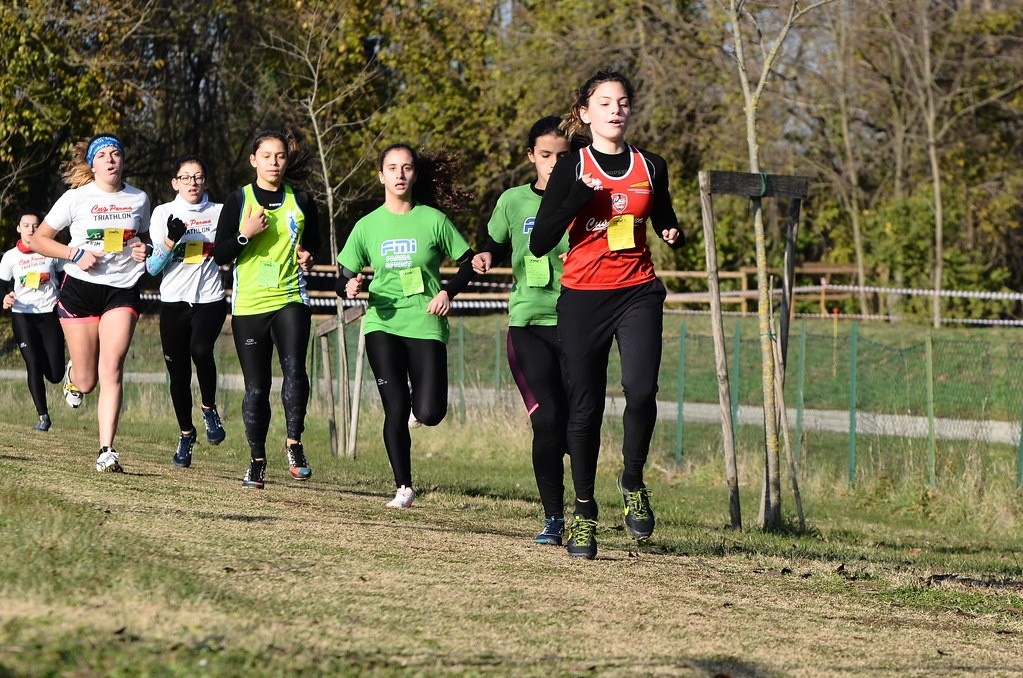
[30,133,154,473]
[529,71,685,559]
[146,155,234,468]
[335,144,477,509]
[471,115,599,546]
[0,212,66,432]
[214,131,321,489]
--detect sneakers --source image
[407,380,422,427]
[173,425,197,468]
[615,473,655,538]
[284,439,311,481]
[386,485,415,509]
[201,405,226,444]
[242,458,267,489]
[536,518,565,545]
[567,513,598,560]
[32,414,52,431]
[96,448,121,473]
[63,358,83,408]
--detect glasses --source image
[175,175,206,185]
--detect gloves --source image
[167,214,188,243]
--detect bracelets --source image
[164,241,173,250]
[236,231,252,246]
[68,247,85,263]
